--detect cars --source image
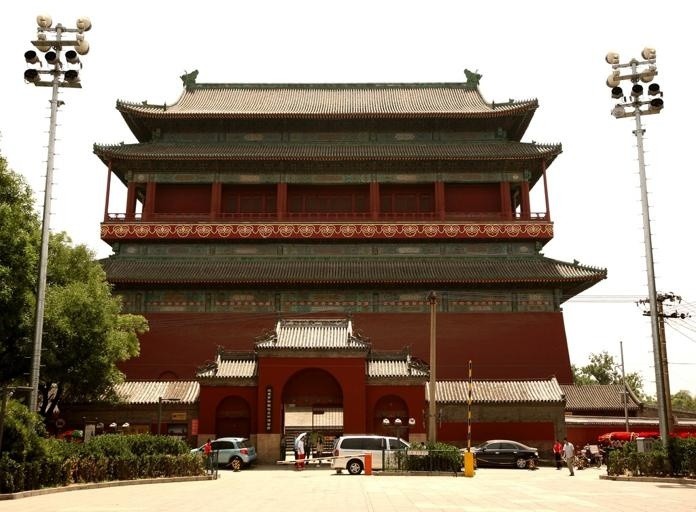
[460,440,539,468]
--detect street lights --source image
[158,396,181,435]
[605,45,681,467]
[612,340,629,433]
[381,418,415,470]
[22,13,95,425]
[94,421,130,435]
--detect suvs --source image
[190,436,258,470]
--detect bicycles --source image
[561,444,603,470]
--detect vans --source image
[331,434,416,474]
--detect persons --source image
[561,437,575,476]
[293,431,323,470]
[205,438,214,472]
[553,438,562,470]
